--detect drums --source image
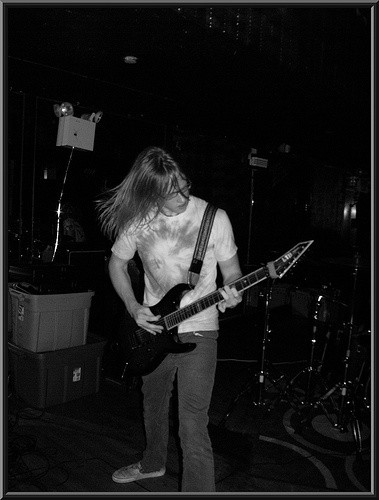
[301,173,371,229]
[289,283,357,323]
[242,283,288,309]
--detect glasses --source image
[164,180,192,200]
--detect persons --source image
[101,147,245,492]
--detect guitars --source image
[108,240,314,377]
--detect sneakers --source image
[112,460,166,483]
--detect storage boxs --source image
[9,333,107,410]
[8,286,94,351]
